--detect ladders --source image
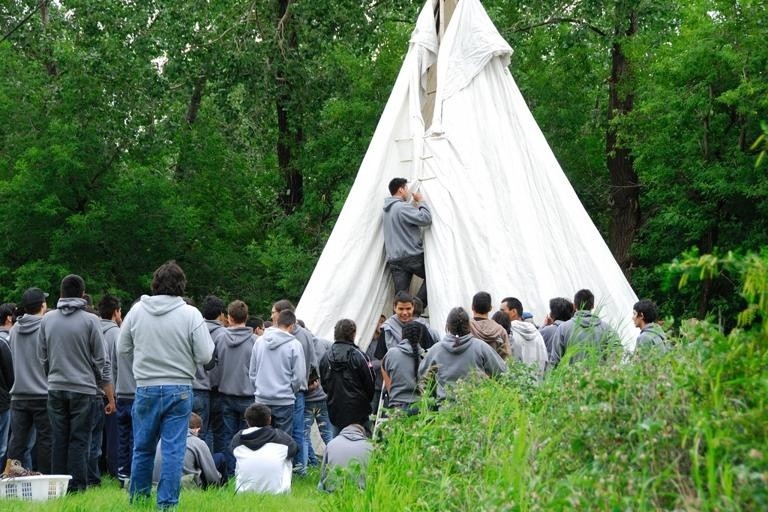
[372,378,396,450]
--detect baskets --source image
[1,472,74,505]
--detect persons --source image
[382,178,432,315]
[116,263,214,507]
[366,289,622,417]
[632,299,670,360]
[2,274,375,495]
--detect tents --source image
[295,0,642,354]
